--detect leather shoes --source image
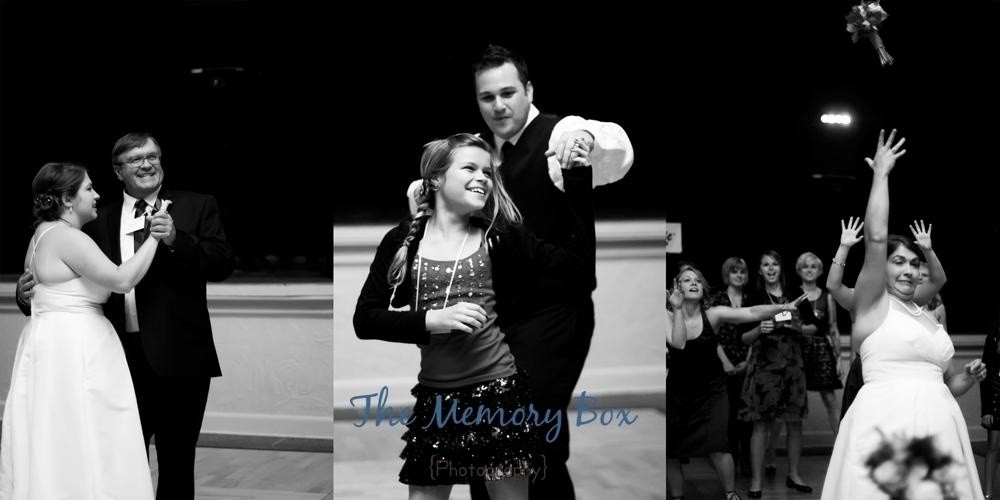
[723,460,776,499]
[786,473,814,493]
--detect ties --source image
[133,200,150,254]
[499,142,514,161]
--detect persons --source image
[352,132,596,500]
[406,43,635,500]
[16,131,235,500]
[666,128,1000,500]
[0,162,168,499]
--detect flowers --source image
[845,2,895,68]
